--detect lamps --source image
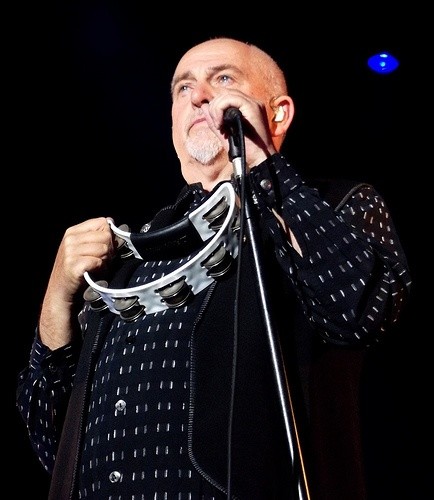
[365,34,401,78]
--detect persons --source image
[15,38,409,500]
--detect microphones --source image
[224,108,242,135]
